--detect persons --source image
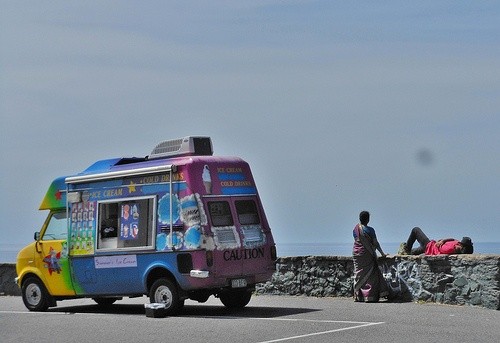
[398,227,473,255]
[353,210,389,302]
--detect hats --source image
[461,237,473,245]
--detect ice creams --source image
[202,165,212,194]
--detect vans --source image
[15,135,277,316]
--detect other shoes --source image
[398,251,404,255]
[401,243,410,255]
